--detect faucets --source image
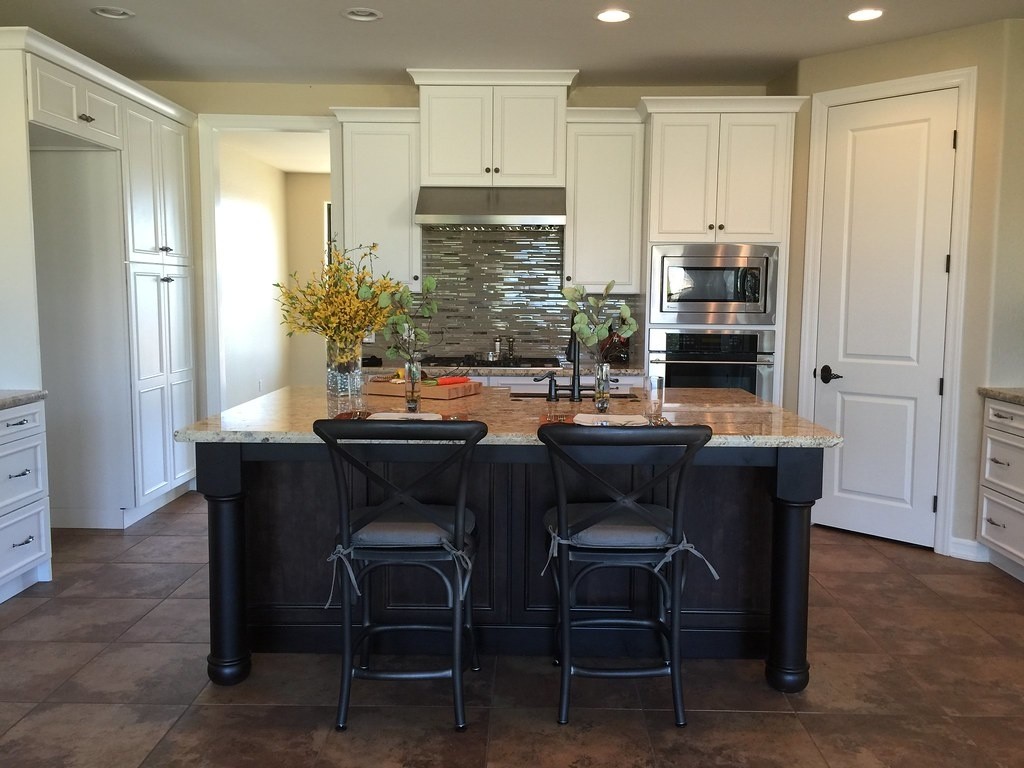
[564,310,582,402]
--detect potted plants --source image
[358,273,439,417]
[558,280,639,415]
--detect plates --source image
[367,413,442,423]
[574,413,649,427]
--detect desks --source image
[173,385,845,693]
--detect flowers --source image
[269,240,405,365]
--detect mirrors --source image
[197,112,348,412]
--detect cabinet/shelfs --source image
[562,105,646,295]
[331,105,422,293]
[0,24,197,529]
[974,397,1024,582]
[642,95,809,244]
[0,399,54,604]
[406,67,578,188]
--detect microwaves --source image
[652,245,779,326]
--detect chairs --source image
[311,418,487,734]
[536,423,711,730]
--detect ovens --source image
[649,327,775,400]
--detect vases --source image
[324,336,362,422]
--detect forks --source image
[558,414,566,423]
[547,413,554,423]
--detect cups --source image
[642,376,663,422]
[348,372,366,411]
